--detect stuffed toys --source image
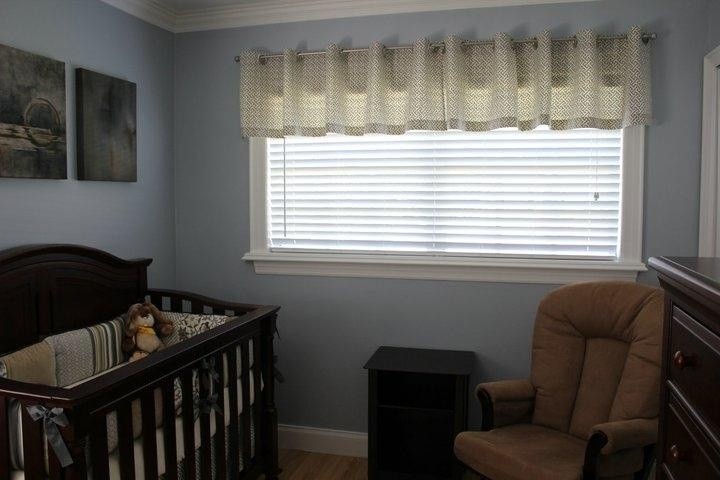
[122,301,175,365]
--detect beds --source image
[1,243,282,480]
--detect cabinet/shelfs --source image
[647,255,720,479]
[363,345,476,480]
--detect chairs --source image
[453,278,666,479]
